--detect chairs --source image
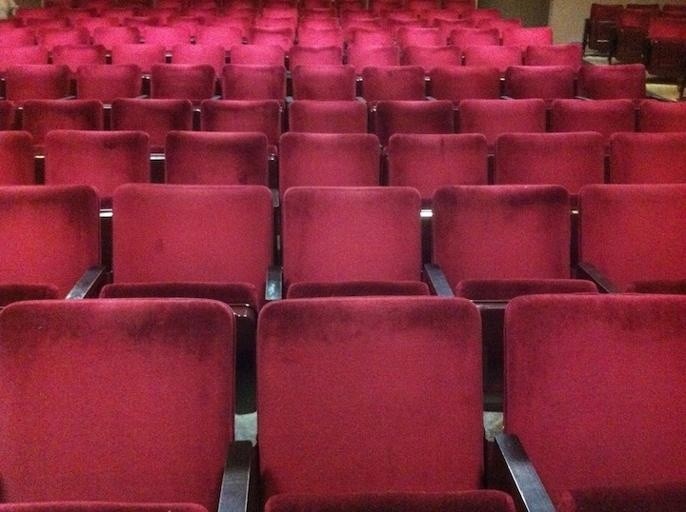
[98,184,280,294]
[576,181,685,294]
[0,298,236,511]
[281,185,425,293]
[253,295,515,512]
[424,184,575,295]
[1,183,98,296]
[492,293,686,511]
[1,1,685,184]
